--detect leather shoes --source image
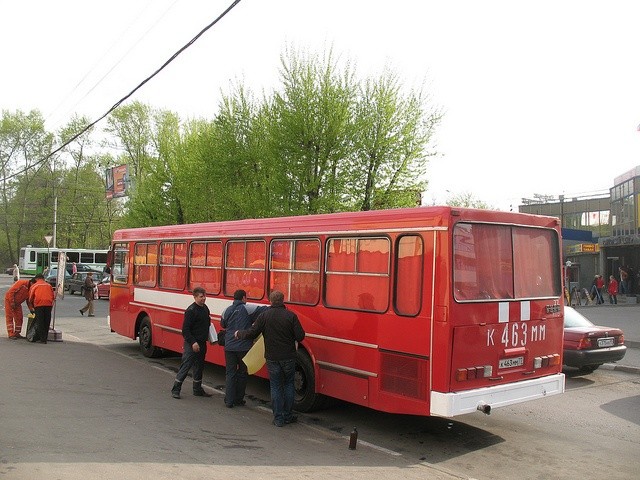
[88,314,95,317]
[80,310,84,316]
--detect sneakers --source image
[272,414,283,427]
[9,336,17,340]
[233,400,246,406]
[17,334,23,338]
[284,413,298,424]
[36,340,47,344]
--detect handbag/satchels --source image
[84,277,92,291]
[14,276,18,281]
[217,329,226,346]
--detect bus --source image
[107,206,565,417]
[19,245,127,274]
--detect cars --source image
[7,266,19,275]
[43,269,71,290]
[562,306,626,377]
[97,275,127,298]
[67,272,103,296]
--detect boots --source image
[193,379,211,397]
[170,379,183,399]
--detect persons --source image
[5,278,34,340]
[171,287,211,399]
[234,290,305,427]
[221,290,270,408]
[618,266,640,294]
[71,262,77,274]
[13,264,20,283]
[592,275,604,304]
[607,275,617,304]
[80,273,96,317]
[28,274,55,343]
[44,266,48,271]
